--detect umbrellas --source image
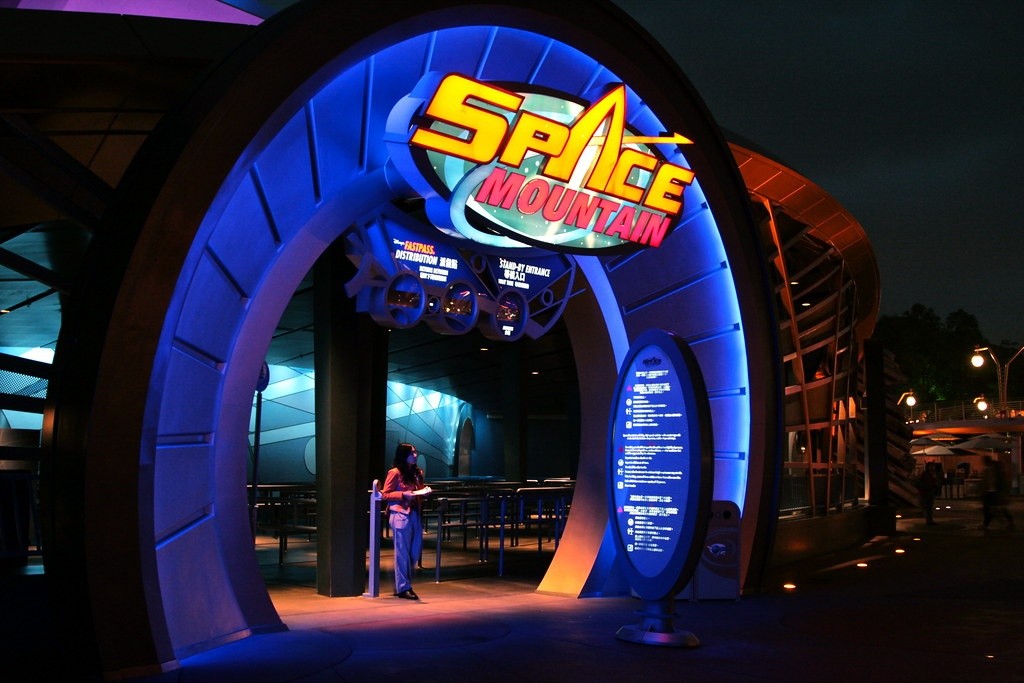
[909,434,1019,463]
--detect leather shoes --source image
[399,589,418,599]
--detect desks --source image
[239,474,580,567]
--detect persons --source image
[974,456,1016,530]
[382,443,433,600]
[919,462,939,526]
